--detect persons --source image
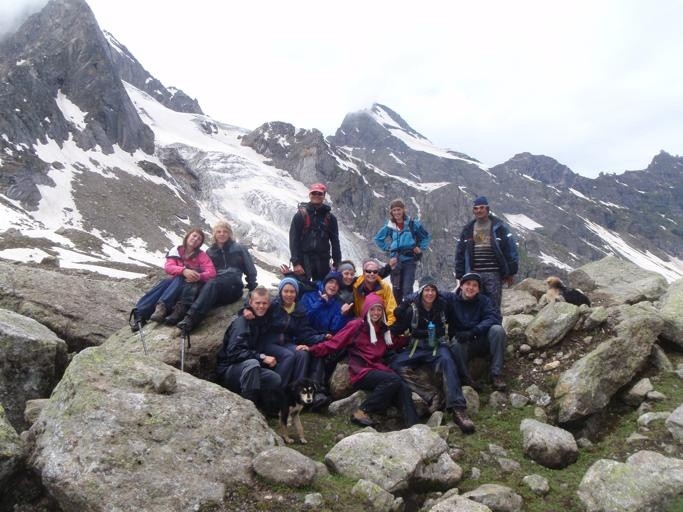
[286,183,341,279]
[387,273,475,433]
[454,196,518,309]
[373,199,432,306]
[163,219,258,334]
[403,273,505,391]
[128,229,217,333]
[296,286,419,428]
[219,258,398,416]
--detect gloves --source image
[454,326,480,344]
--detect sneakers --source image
[176,315,196,332]
[165,305,185,324]
[131,312,146,332]
[312,386,327,407]
[350,406,377,427]
[452,408,475,434]
[464,378,482,393]
[427,389,446,414]
[149,303,167,323]
[490,372,507,391]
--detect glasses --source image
[364,270,380,275]
[473,206,486,210]
[309,191,324,196]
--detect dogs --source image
[543,276,591,307]
[250,377,319,444]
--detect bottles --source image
[427,323,436,347]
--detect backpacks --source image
[414,234,423,261]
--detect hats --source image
[308,183,326,194]
[388,200,404,210]
[278,277,299,294]
[418,275,438,295]
[363,258,378,274]
[472,195,487,206]
[459,273,481,287]
[322,272,343,288]
[336,261,354,274]
